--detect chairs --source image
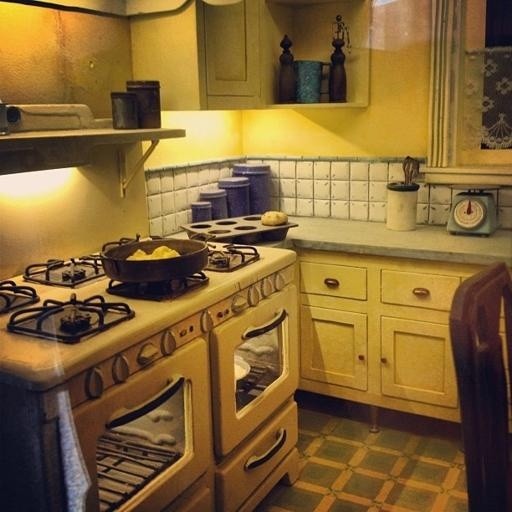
[448,260,511,512]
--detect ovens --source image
[45,285,301,512]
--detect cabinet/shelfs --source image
[287,250,512,424]
[128,0,371,111]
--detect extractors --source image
[0,104,188,176]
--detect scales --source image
[447,183,502,236]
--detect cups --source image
[110,78,162,129]
[294,59,331,103]
[388,183,420,232]
[191,161,270,222]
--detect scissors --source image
[403,156,419,186]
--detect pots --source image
[98,238,210,281]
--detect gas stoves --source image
[1,235,298,419]
